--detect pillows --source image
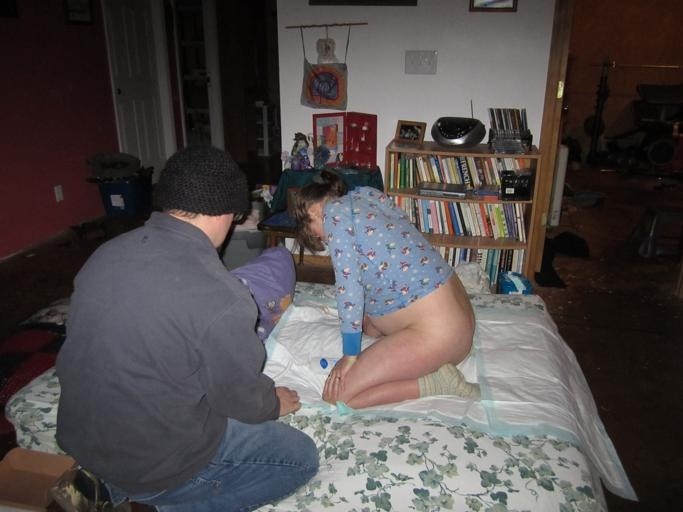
[227,242,297,342]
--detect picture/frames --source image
[395,119,427,146]
[469,0,517,13]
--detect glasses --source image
[233,212,245,221]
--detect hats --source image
[153,145,249,216]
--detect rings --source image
[335,376,340,380]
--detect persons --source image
[48,144,319,510]
[274,167,481,410]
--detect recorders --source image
[430,116,486,146]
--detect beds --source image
[0,244,608,512]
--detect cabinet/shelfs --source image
[384,136,543,292]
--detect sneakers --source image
[50,464,157,511]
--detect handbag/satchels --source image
[300,57,347,109]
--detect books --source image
[384,107,537,297]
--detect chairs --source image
[258,170,312,267]
